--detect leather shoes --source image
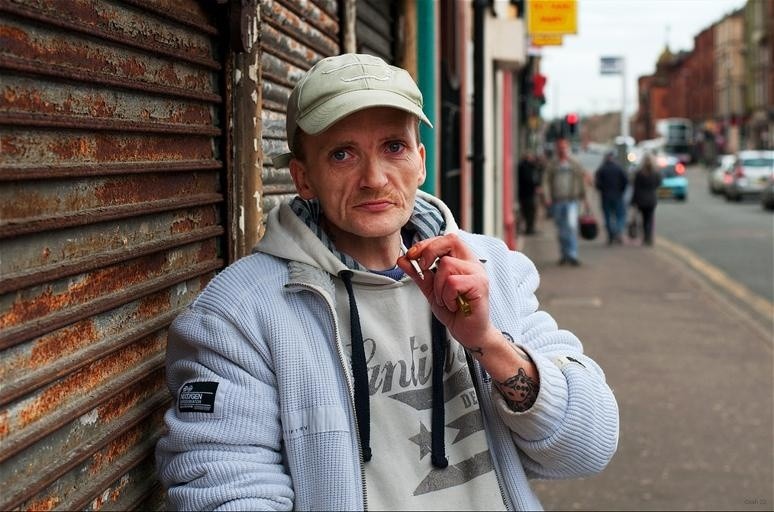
[567,255,578,266]
[559,256,568,264]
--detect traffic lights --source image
[564,113,579,140]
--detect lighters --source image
[456,295,473,319]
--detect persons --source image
[157,51,621,512]
[631,152,662,247]
[541,137,592,268]
[517,151,541,234]
[596,151,630,244]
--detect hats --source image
[284,53,433,156]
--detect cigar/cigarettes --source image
[399,243,426,281]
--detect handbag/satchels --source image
[580,204,596,239]
[629,221,636,239]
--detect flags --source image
[531,35,564,48]
[528,0,580,36]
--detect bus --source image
[652,116,697,163]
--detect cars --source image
[586,138,774,204]
